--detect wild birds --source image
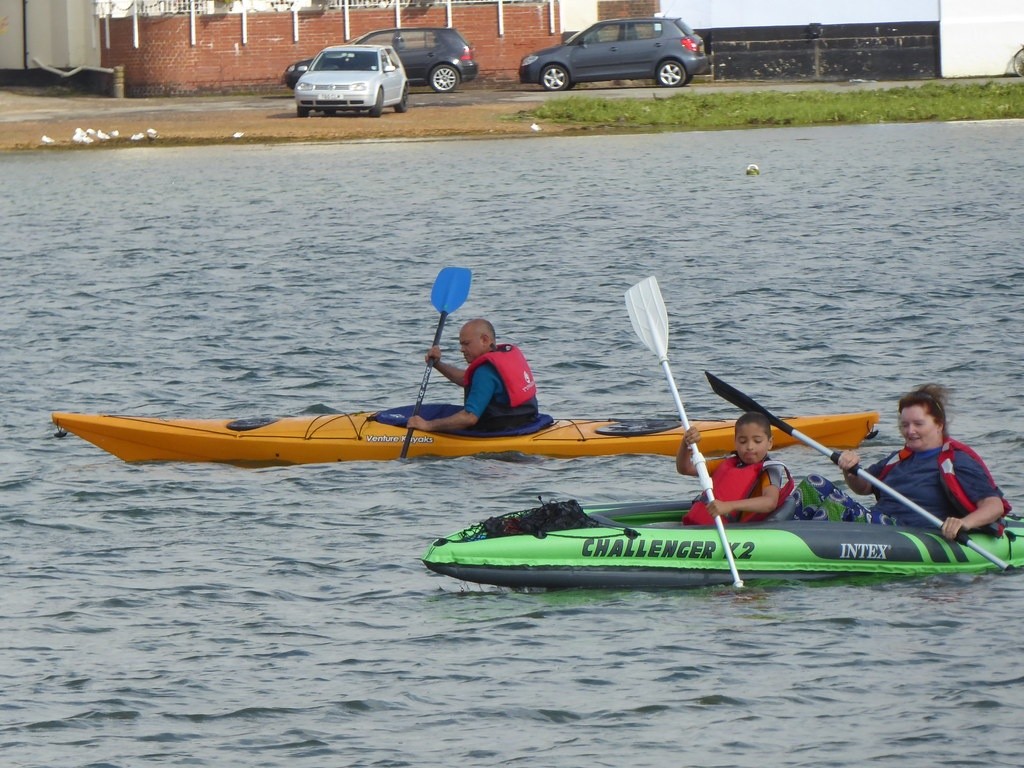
[41,135,56,146]
[530,122,546,133]
[70,127,160,145]
[232,131,246,138]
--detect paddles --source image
[702,369,1016,572]
[622,271,749,592]
[398,264,472,461]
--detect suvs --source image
[284,27,479,95]
[519,16,706,92]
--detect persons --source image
[407,319,539,432]
[676,413,795,525]
[838,385,1012,540]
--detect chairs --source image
[626,27,637,39]
[765,494,799,521]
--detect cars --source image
[295,45,409,117]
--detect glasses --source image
[916,391,943,413]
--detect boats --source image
[420,502,1024,590]
[53,413,878,465]
